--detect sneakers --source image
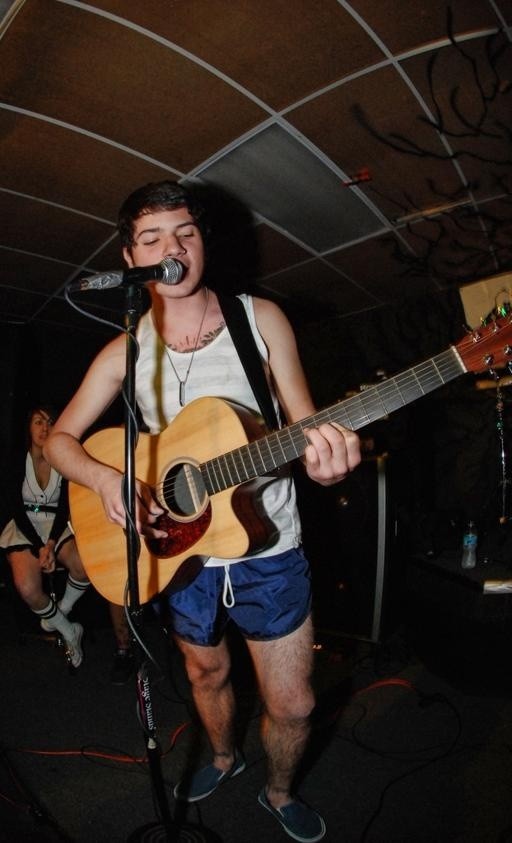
[109,648,132,686]
[173,754,245,802]
[41,609,83,667]
[257,787,326,843]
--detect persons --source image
[0,402,91,669]
[43,179,363,842]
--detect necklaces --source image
[153,287,209,408]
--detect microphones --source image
[82,258,186,291]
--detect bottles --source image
[460,520,478,568]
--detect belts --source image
[24,504,57,512]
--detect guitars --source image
[68,304,512,608]
